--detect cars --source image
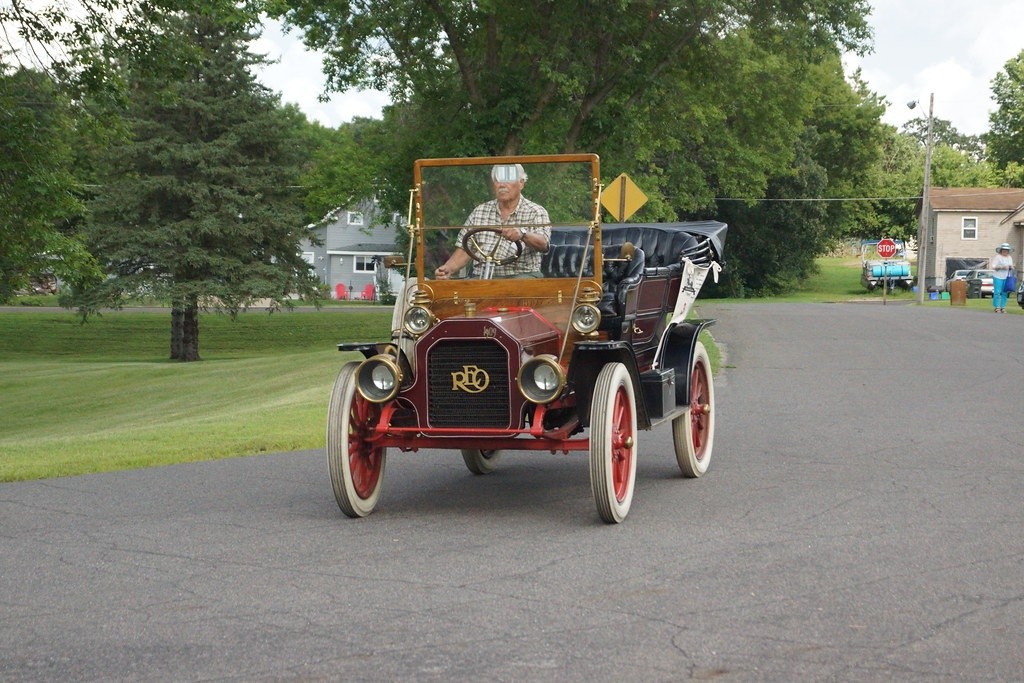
[946,269,995,298]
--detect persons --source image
[435,164,552,280]
[991,243,1015,313]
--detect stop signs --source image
[876,238,896,258]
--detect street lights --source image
[907,101,933,305]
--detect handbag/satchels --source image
[1004,266,1017,292]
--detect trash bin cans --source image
[950,280,967,306]
[967,278,982,299]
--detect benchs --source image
[551,226,699,269]
[541,242,646,318]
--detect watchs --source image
[520,228,526,240]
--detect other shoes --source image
[994,308,1007,314]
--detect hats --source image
[996,243,1014,255]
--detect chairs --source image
[336,281,348,300]
[360,284,374,300]
